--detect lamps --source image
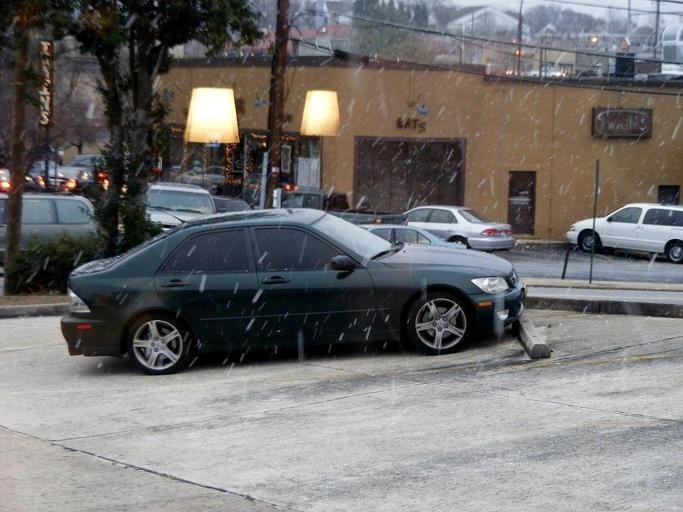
[184,87,241,145]
[300,90,341,137]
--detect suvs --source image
[145,181,217,232]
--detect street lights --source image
[183,83,240,185]
[296,89,341,189]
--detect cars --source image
[360,223,467,250]
[55,203,531,381]
[0,144,262,213]
[1,191,102,263]
[401,204,515,254]
[560,200,682,265]
[530,54,682,85]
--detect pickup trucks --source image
[281,188,406,226]
